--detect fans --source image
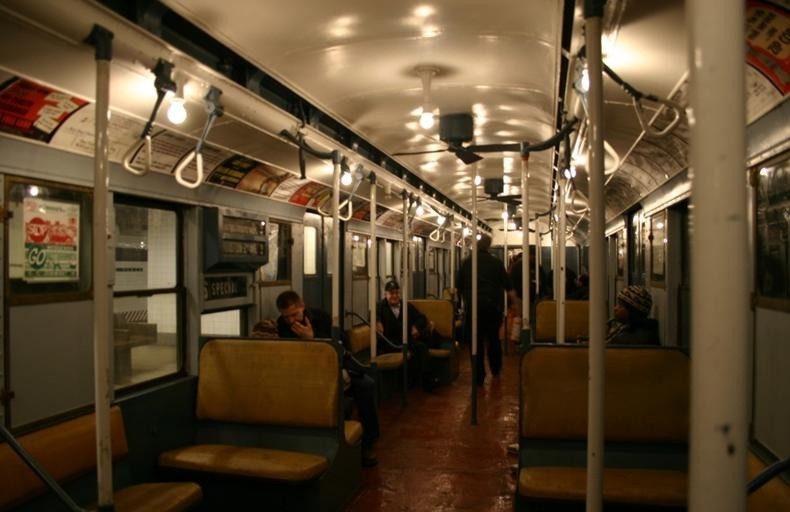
[475,177,522,207]
[390,113,530,168]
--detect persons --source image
[505,247,589,318]
[457,233,510,388]
[276,291,380,466]
[251,320,278,339]
[508,286,652,467]
[366,282,440,392]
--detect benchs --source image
[348,320,405,397]
[511,299,688,509]
[158,337,361,481]
[408,301,452,379]
[5,406,201,510]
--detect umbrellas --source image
[362,448,378,466]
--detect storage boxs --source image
[511,299,688,509]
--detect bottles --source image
[617,285,653,316]
[385,282,399,291]
[251,319,277,337]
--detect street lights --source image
[418,329,459,392]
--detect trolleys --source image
[475,177,522,207]
[390,113,530,168]
